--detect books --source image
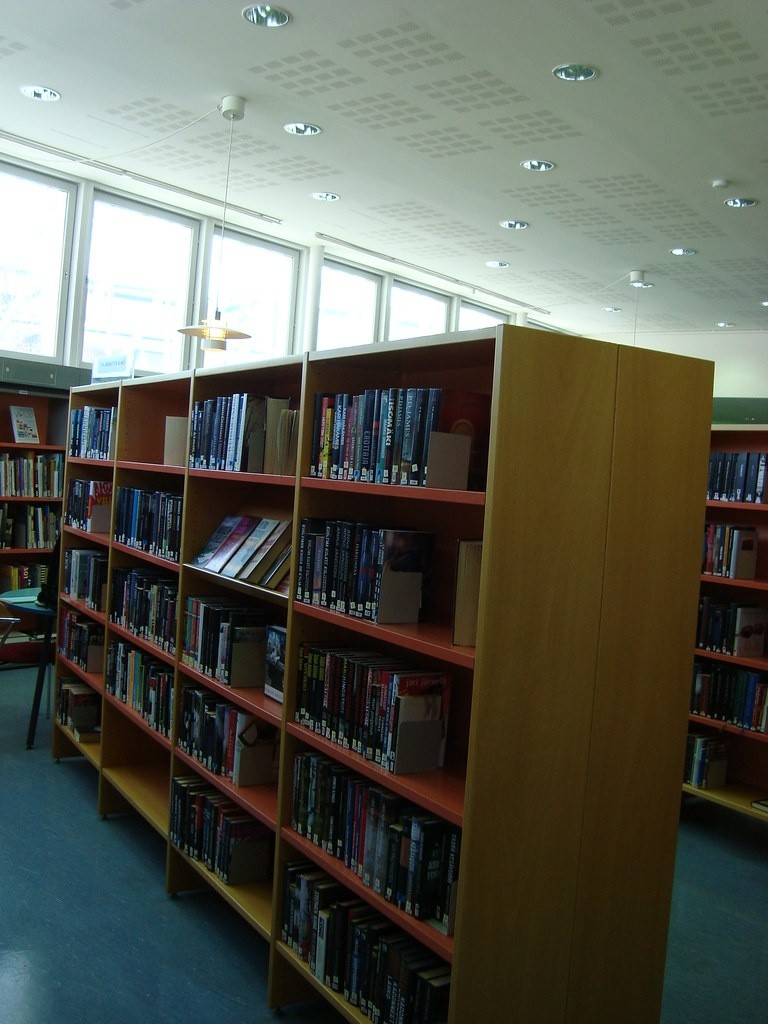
[189,393,299,477]
[189,515,292,594]
[0,405,65,593]
[310,386,491,491]
[681,452,768,815]
[281,856,450,1024]
[69,406,118,461]
[294,518,482,647]
[57,416,189,743]
[176,682,280,786]
[181,595,287,705]
[169,773,275,885]
[290,751,461,938]
[293,643,450,775]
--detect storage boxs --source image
[0,349,93,391]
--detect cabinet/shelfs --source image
[49,323,714,1024]
[0,386,70,669]
[683,423,768,822]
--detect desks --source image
[0,587,58,749]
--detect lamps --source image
[175,92,252,349]
